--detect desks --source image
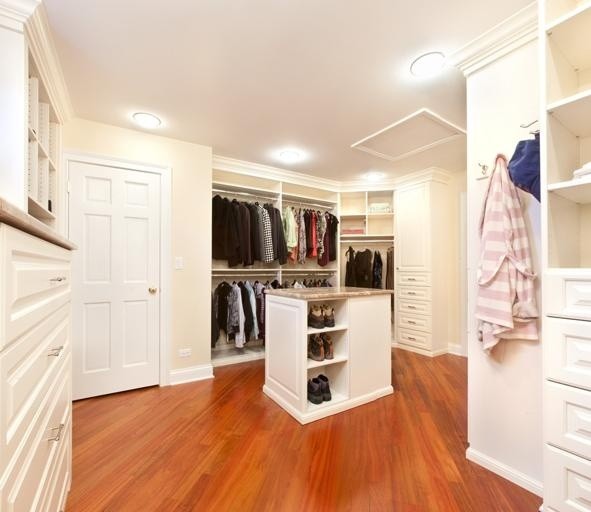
[263,287,394,425]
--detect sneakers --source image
[320,303,337,327]
[317,374,331,401]
[307,304,325,329]
[318,333,334,359]
[306,333,325,362]
[307,377,323,404]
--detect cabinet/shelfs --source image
[0,0,76,512]
[339,180,394,287]
[281,169,340,287]
[539,0,591,512]
[394,166,449,357]
[211,155,281,367]
